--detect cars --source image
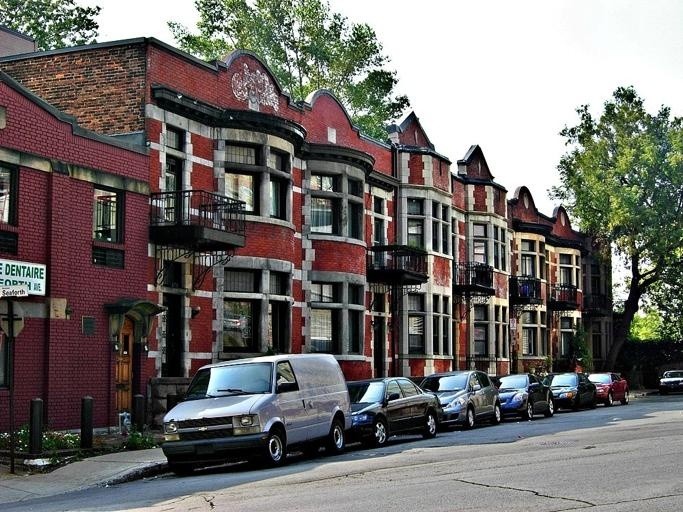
[657,370,682,394]
[417,369,503,432]
[540,372,599,414]
[340,376,445,452]
[584,371,630,409]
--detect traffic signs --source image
[0,286,28,298]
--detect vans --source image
[159,354,354,481]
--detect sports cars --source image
[491,372,555,423]
